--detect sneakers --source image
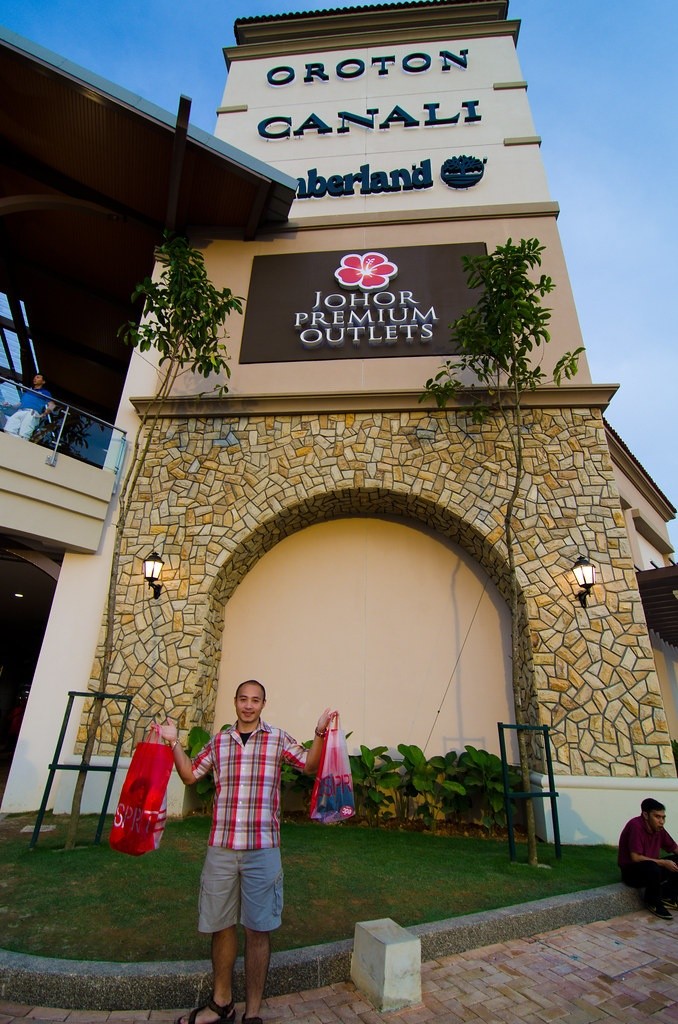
[659,896,678,909]
[647,903,674,919]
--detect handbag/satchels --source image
[110,725,175,856]
[310,712,356,823]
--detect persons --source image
[618,798,678,919]
[150,680,338,1024]
[3,373,54,440]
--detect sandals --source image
[175,996,236,1024]
[242,1013,263,1024]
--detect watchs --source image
[315,726,327,738]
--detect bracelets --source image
[172,738,180,750]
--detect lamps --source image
[142,551,165,599]
[571,556,596,610]
